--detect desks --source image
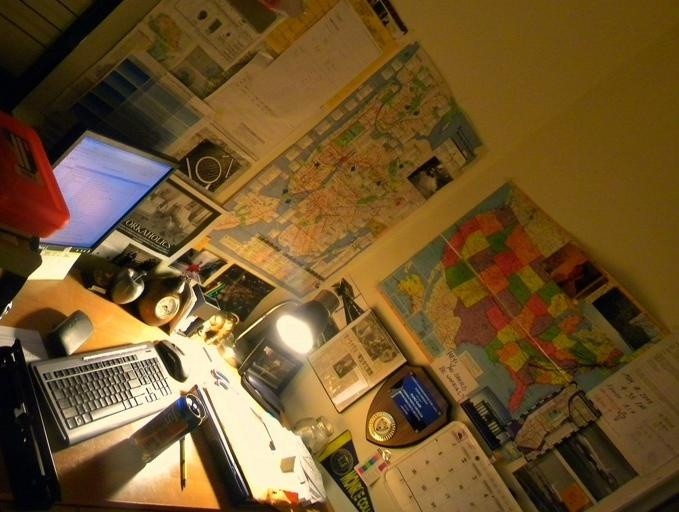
[0,265,334,512]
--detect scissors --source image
[212,368,229,391]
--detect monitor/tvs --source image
[38,128,179,254]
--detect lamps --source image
[207,288,340,369]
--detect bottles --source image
[291,416,335,458]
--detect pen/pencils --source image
[203,347,212,362]
[180,436,187,488]
[205,281,226,296]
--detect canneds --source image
[129,391,206,465]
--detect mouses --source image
[154,340,187,382]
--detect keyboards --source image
[29,341,182,450]
